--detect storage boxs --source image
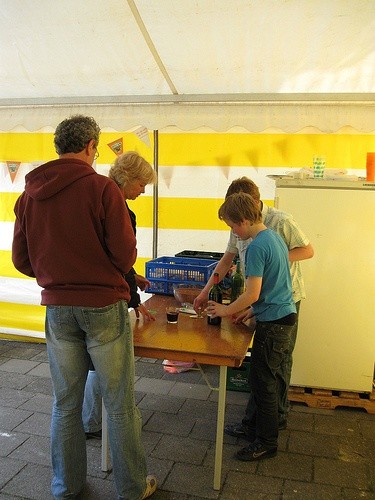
[226,348,255,392]
[145,255,219,296]
[176,250,226,261]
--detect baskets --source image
[145,255,219,295]
[175,251,240,266]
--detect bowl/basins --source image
[173,288,208,307]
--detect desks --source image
[100,292,255,490]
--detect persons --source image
[11,115,157,500]
[81,152,155,438]
[193,177,314,430]
[205,192,298,461]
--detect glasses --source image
[94,146,100,159]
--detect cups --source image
[166,307,179,324]
[366,152,375,183]
[312,156,325,179]
[231,310,244,325]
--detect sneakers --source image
[225,422,254,441]
[235,442,278,462]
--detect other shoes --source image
[140,475,156,500]
[84,429,102,439]
[279,425,288,430]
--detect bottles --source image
[207,272,222,325]
[230,258,244,304]
